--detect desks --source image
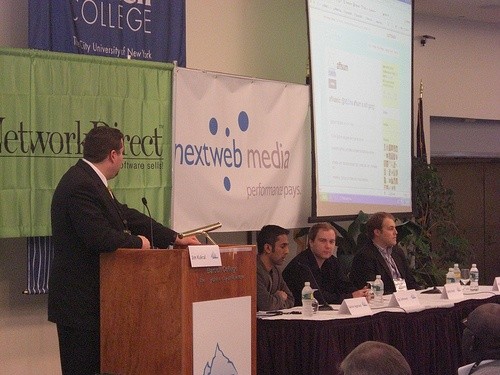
[256,285,500,375]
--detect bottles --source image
[374,275,383,306]
[446,268,455,293]
[470,264,479,291]
[301,282,313,318]
[454,263,461,291]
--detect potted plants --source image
[408,155,471,284]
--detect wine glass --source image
[460,269,470,292]
[311,289,320,314]
[366,281,375,305]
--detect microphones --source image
[142,197,154,249]
[411,251,442,294]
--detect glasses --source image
[462,318,476,334]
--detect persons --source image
[46,127,201,375]
[282,222,369,304]
[353,211,415,296]
[256,224,293,312]
[340,341,412,375]
[458,303,500,375]
